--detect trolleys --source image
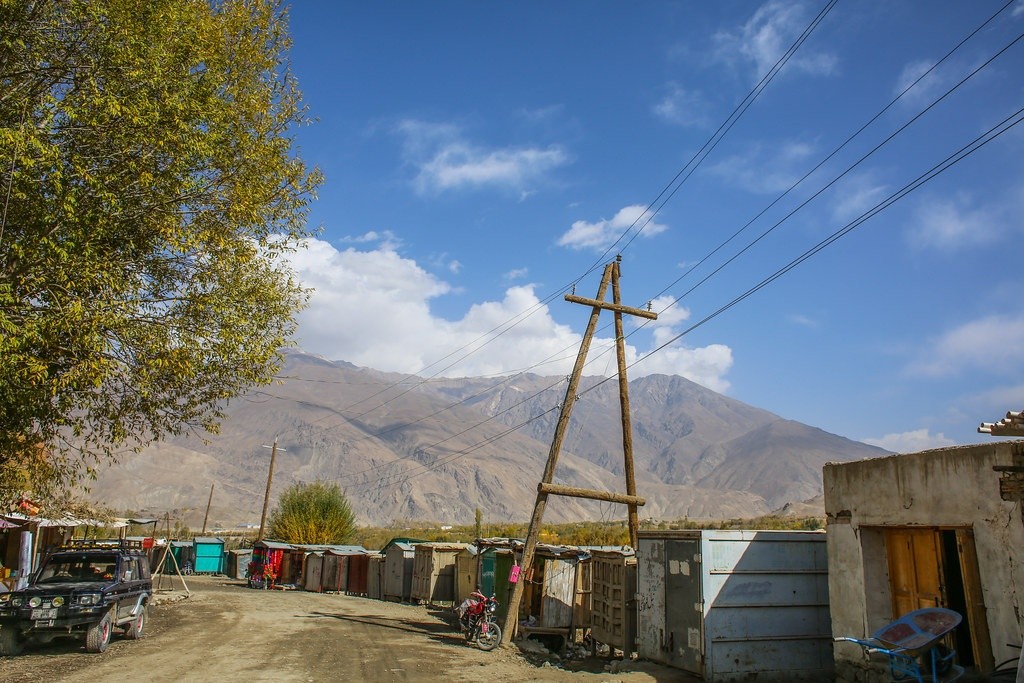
[833,607,962,683]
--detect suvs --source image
[0,538,153,656]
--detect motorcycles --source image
[460,589,501,651]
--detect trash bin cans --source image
[153,546,182,573]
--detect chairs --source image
[68,567,95,577]
[106,565,116,576]
